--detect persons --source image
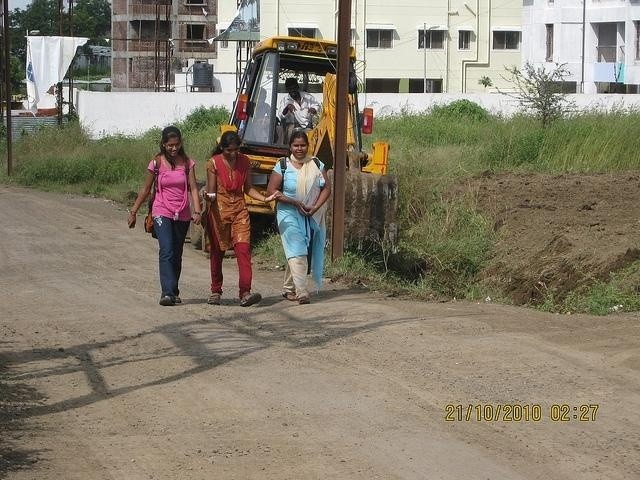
[126,125,203,306]
[241,85,271,124]
[265,131,332,305]
[275,76,322,130]
[203,130,283,307]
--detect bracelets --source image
[130,211,136,216]
[264,197,266,202]
[193,211,200,215]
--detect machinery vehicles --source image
[191,35,389,253]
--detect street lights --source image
[424,23,440,93]
[26,30,40,36]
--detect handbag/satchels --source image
[144,209,154,233]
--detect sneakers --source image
[283,292,297,300]
[160,295,176,306]
[297,292,310,304]
[207,292,221,305]
[176,296,181,303]
[240,293,261,307]
